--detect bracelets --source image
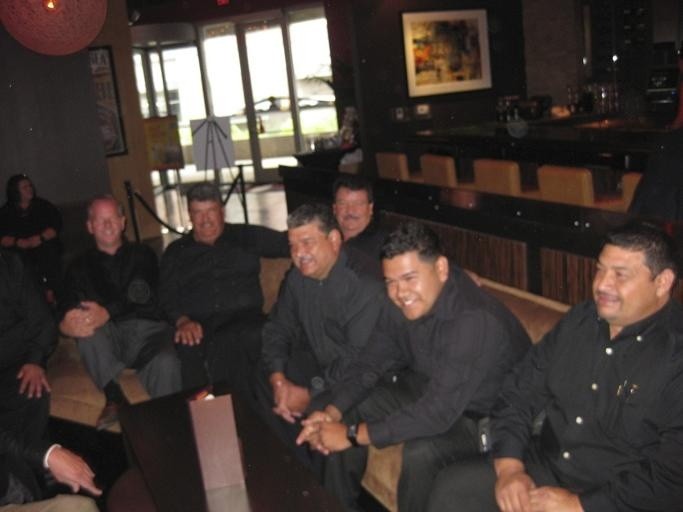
[274,379,288,387]
[13,235,22,248]
[319,409,337,421]
[38,232,46,245]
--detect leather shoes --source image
[96,396,131,432]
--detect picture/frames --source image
[87,45,129,157]
[398,7,495,98]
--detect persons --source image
[0,175,65,276]
[426,222,680,510]
[54,190,181,429]
[0,427,135,510]
[293,217,534,511]
[251,195,407,512]
[160,178,266,395]
[272,174,396,324]
[1,248,54,439]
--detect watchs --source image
[345,419,361,446]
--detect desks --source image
[276,163,683,317]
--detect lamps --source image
[0,0,108,57]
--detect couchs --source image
[356,273,574,512]
[43,257,291,427]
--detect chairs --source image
[421,155,458,188]
[539,166,594,207]
[473,159,521,197]
[374,153,410,182]
[622,172,644,212]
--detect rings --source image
[84,317,92,325]
